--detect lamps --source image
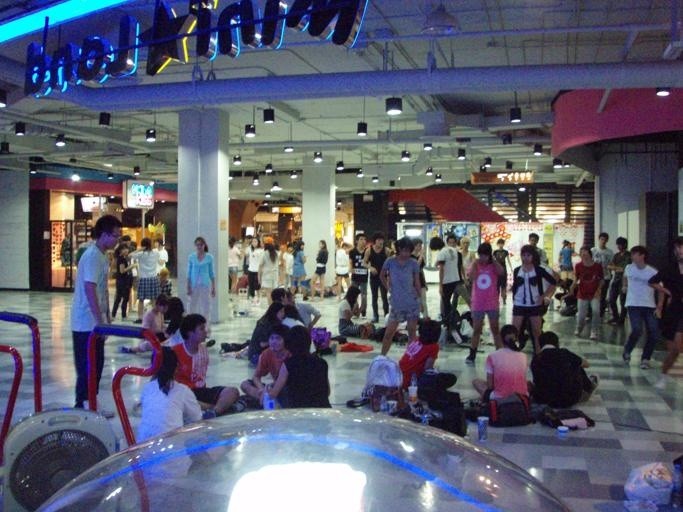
[385,96,403,115]
[230,109,297,207]
[311,152,381,184]
[357,121,369,137]
[389,143,442,189]
[509,107,522,124]
[421,3,459,35]
[655,88,670,97]
[455,134,572,192]
[1,112,157,181]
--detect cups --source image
[476,416,489,442]
[407,386,418,404]
[386,400,396,415]
[555,426,568,440]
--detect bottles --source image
[380,394,387,416]
[410,372,418,387]
[668,465,682,508]
[261,392,274,410]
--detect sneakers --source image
[574,324,585,336]
[228,400,246,412]
[590,328,600,338]
[465,345,477,365]
[639,359,651,370]
[590,373,600,389]
[519,334,529,350]
[623,343,632,364]
[119,345,136,354]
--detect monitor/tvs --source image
[81,196,106,212]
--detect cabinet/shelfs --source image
[76,225,91,250]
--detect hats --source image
[263,237,274,244]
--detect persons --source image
[530,331,596,405]
[186,236,217,338]
[622,246,664,369]
[229,237,328,306]
[111,235,171,323]
[172,313,247,418]
[71,215,122,418]
[647,236,682,390]
[248,288,321,366]
[138,346,202,444]
[430,235,474,319]
[399,319,457,390]
[120,294,184,354]
[472,324,532,402]
[466,243,504,362]
[379,237,421,357]
[512,233,560,341]
[555,233,632,339]
[493,239,512,306]
[240,324,332,408]
[336,234,427,340]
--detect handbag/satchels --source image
[541,406,594,431]
[487,392,537,428]
[346,385,404,414]
